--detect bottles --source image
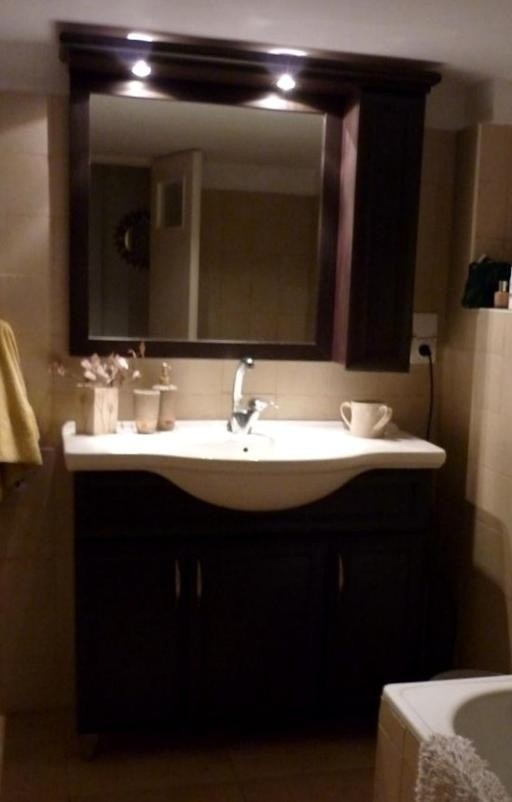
[150,360,179,432]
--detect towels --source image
[1,317,44,466]
[413,728,511,801]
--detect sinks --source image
[127,413,371,466]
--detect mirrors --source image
[83,91,327,348]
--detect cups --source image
[132,389,162,433]
[339,401,396,438]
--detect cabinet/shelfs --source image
[74,521,321,737]
[323,524,435,732]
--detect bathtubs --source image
[371,676,512,796]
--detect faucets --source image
[233,356,265,437]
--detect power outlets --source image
[408,337,438,366]
